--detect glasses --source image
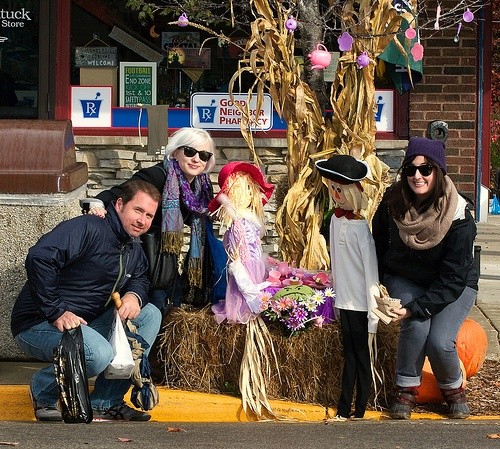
[402,163,433,177]
[177,146,213,162]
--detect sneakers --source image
[30,383,63,421]
[388,388,415,420]
[93,400,151,422]
[440,385,470,419]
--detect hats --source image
[208,161,275,212]
[404,137,447,176]
[314,155,368,183]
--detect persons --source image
[315,154,380,422]
[11,181,162,422]
[208,162,274,420]
[79,128,215,309]
[372,137,477,420]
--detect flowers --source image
[261,286,335,332]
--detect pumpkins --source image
[414,356,469,404]
[454,317,488,378]
